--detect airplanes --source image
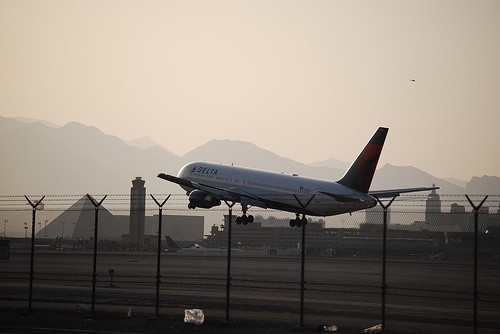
[164,235,246,257]
[155,126,440,228]
